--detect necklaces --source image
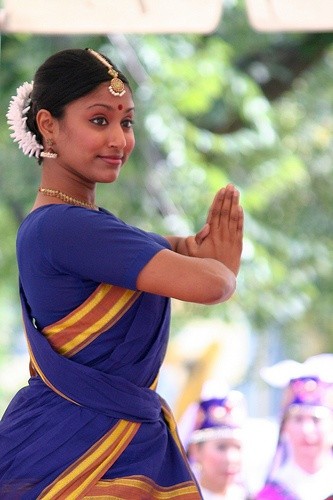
[40,189,98,210]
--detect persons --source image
[246,355,333,499]
[186,383,252,500]
[0,48,243,499]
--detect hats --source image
[286,377,332,418]
[191,398,243,443]
